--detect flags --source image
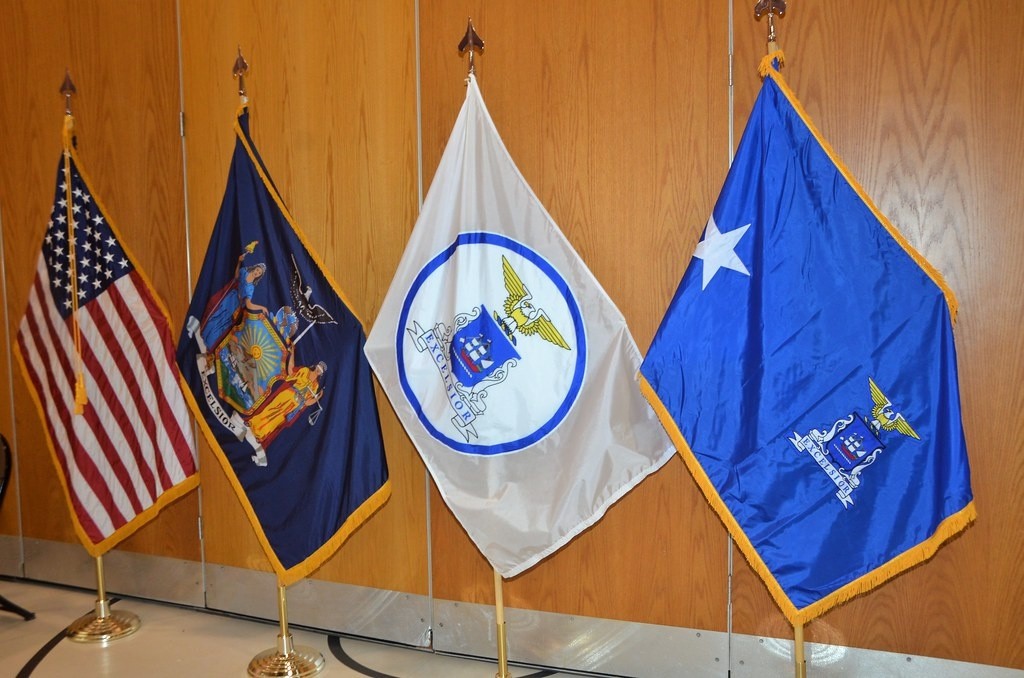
[633,43,977,631]
[10,115,200,562]
[363,75,677,583]
[173,101,393,587]
